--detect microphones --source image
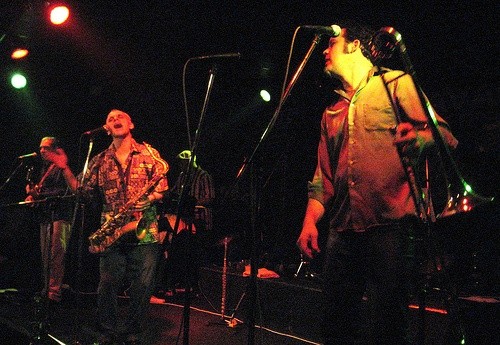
[84,126,109,135]
[300,24,341,38]
[191,53,240,62]
[18,152,38,158]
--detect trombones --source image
[368,25,496,344]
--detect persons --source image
[24,136,75,310]
[165,150,215,304]
[42,106,171,345]
[296,20,459,345]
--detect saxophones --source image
[87,172,166,254]
